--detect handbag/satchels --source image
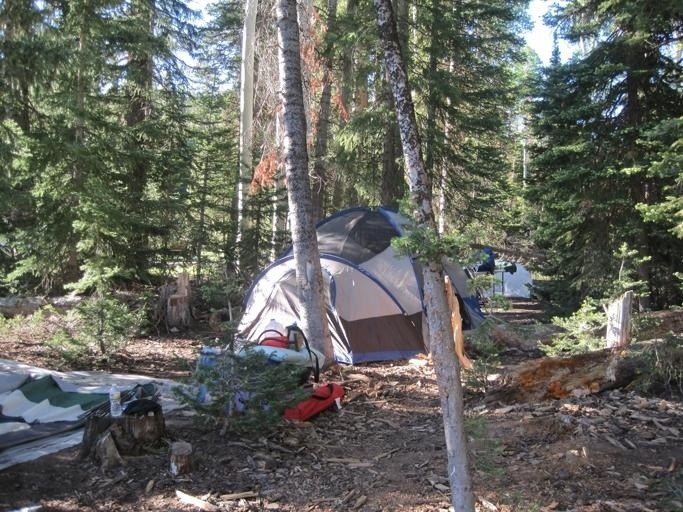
[284,383,344,421]
[261,325,304,351]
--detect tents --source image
[229,206,486,362]
[462,247,533,300]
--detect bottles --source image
[108,383,124,418]
[196,381,208,403]
